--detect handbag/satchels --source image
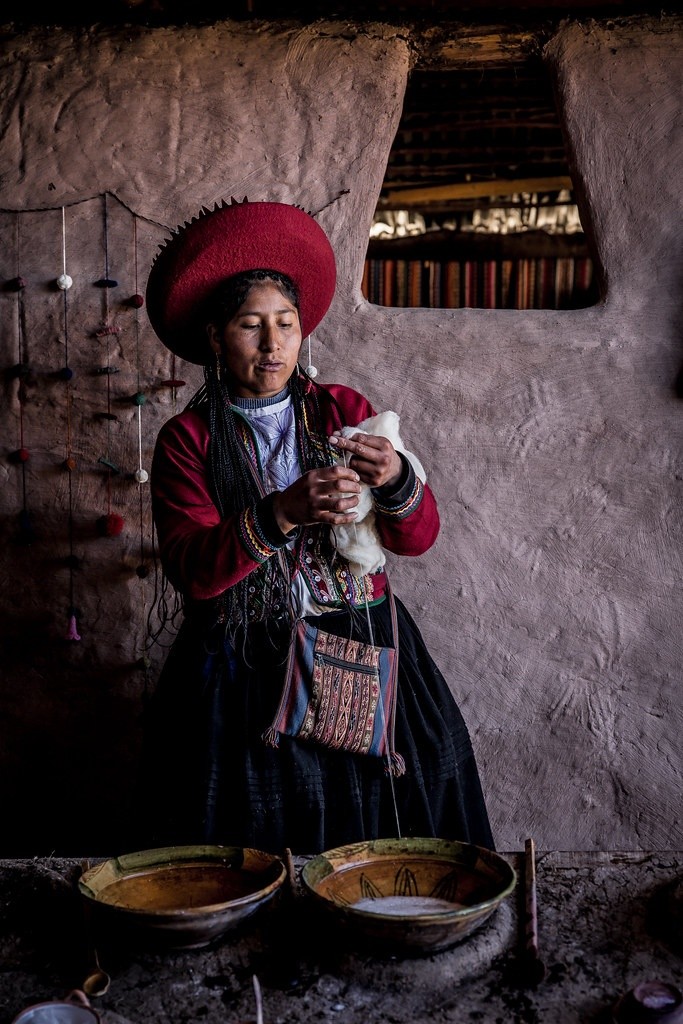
[264,617,399,768]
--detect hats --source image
[146,195,338,365]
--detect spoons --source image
[80,861,111,998]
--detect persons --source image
[149,195,502,858]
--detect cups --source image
[11,989,101,1024]
[630,980,681,1023]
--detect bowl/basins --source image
[77,846,287,950]
[300,838,516,956]
